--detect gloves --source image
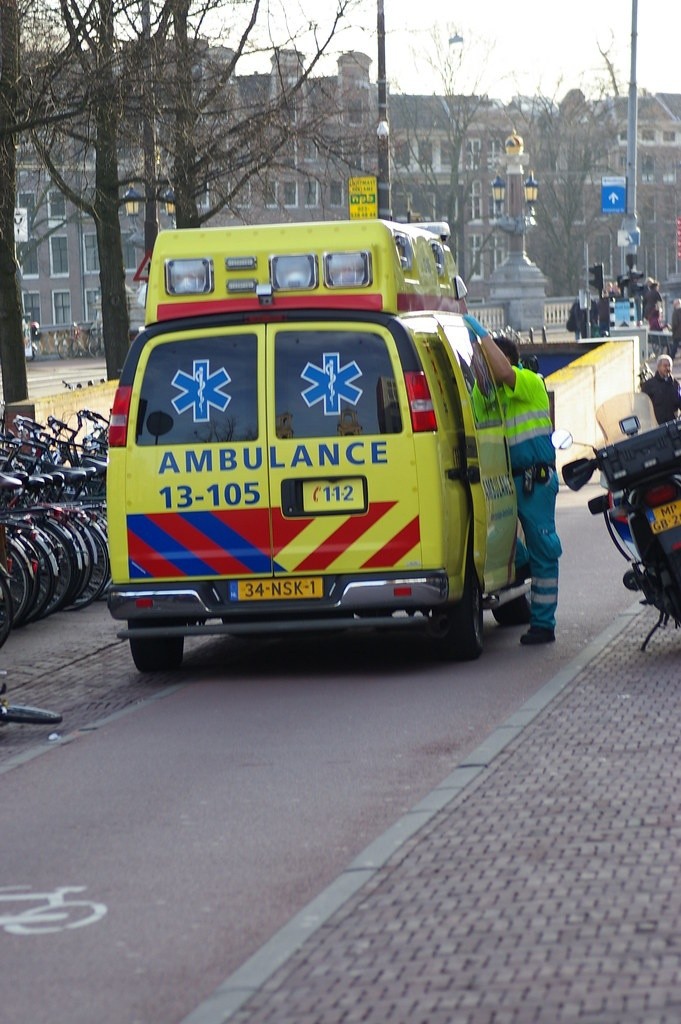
[464,314,488,337]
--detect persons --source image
[464,313,562,645]
[666,299,681,358]
[567,295,598,340]
[466,326,501,430]
[599,277,662,337]
[641,354,681,425]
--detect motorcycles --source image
[551,391,681,653]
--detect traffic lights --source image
[631,270,644,293]
[589,263,604,289]
[617,275,630,288]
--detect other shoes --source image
[519,626,556,645]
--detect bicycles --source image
[0,409,112,648]
[57,324,106,359]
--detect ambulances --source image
[103,220,532,678]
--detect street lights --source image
[491,130,539,265]
